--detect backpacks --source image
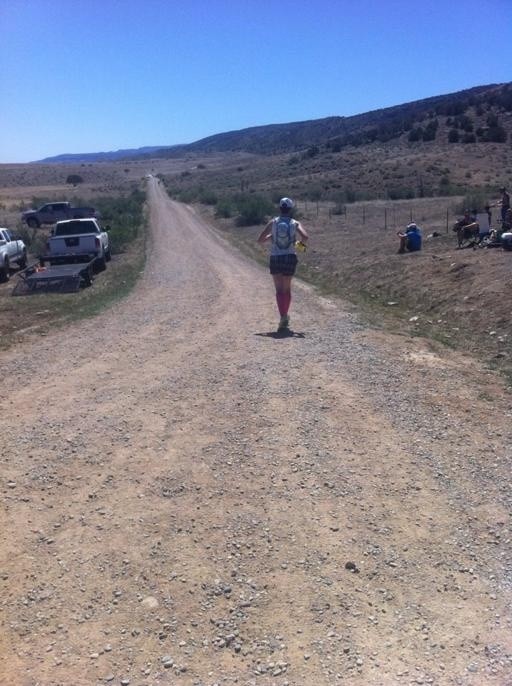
[273,218,293,250]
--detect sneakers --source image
[279,315,289,330]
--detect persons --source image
[453,208,479,245]
[396,223,422,252]
[496,186,510,229]
[257,196,308,330]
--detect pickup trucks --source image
[0,226,28,283]
[20,201,96,228]
[45,215,112,269]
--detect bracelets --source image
[300,241,305,246]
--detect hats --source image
[280,197,294,209]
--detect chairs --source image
[457,218,478,247]
